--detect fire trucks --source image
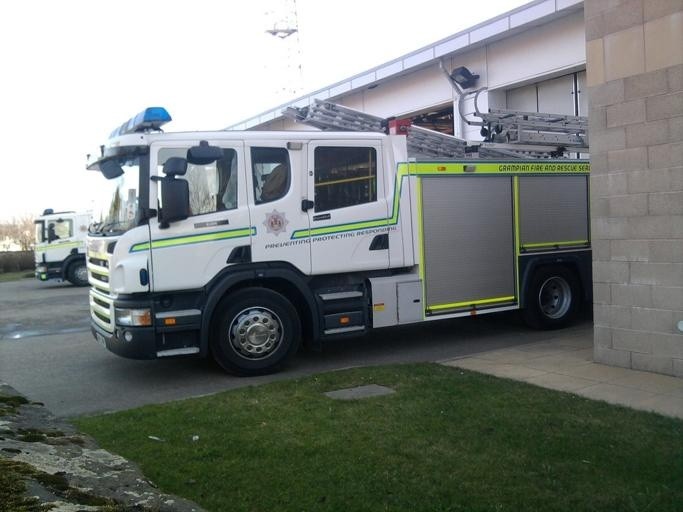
[31,208,90,289]
[83,84,591,378]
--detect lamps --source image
[449,66,480,89]
